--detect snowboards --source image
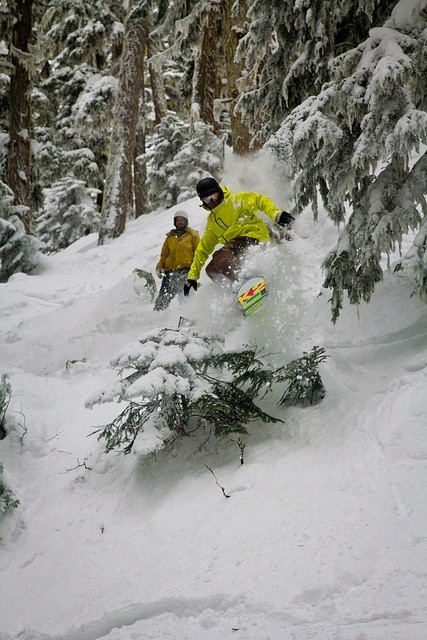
[237,277,268,320]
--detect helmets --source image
[196,178,221,199]
[174,210,188,219]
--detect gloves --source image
[277,211,295,227]
[156,270,162,278]
[184,279,197,296]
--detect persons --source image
[151,209,200,312]
[182,177,295,294]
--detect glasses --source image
[201,192,220,204]
